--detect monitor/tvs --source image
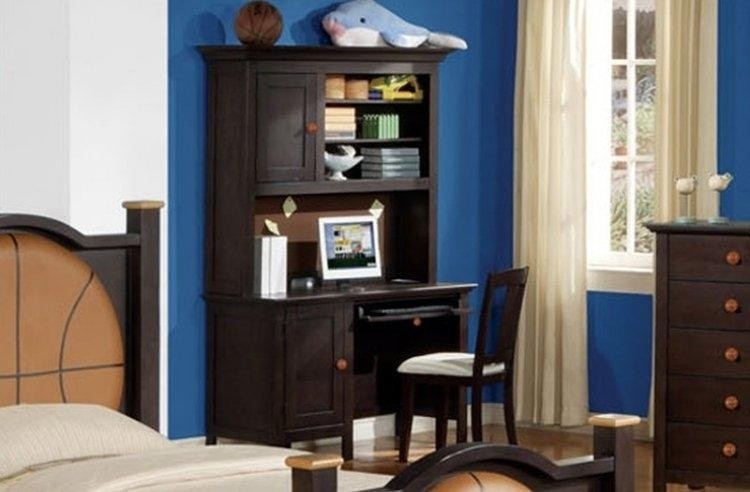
[318,215,383,291]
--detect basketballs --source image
[235,1,283,46]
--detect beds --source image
[0,201,640,489]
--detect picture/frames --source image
[316,214,386,282]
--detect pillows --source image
[2,402,171,482]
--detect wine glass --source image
[708,173,732,224]
[674,175,697,224]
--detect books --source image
[360,146,421,178]
[324,107,358,140]
[255,236,287,298]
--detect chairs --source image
[400,261,532,461]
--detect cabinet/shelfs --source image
[189,43,478,461]
[642,223,748,491]
